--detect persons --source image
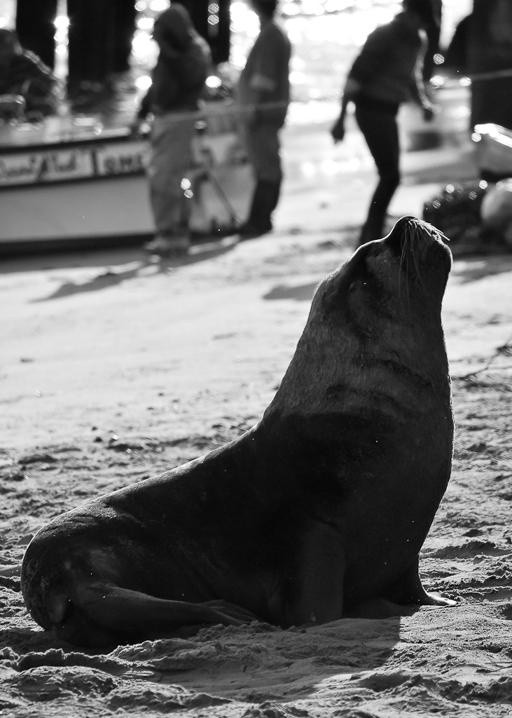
[125,6,212,256]
[1,4,231,121]
[440,0,510,180]
[236,0,293,234]
[326,1,434,248]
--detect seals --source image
[19,215,455,657]
